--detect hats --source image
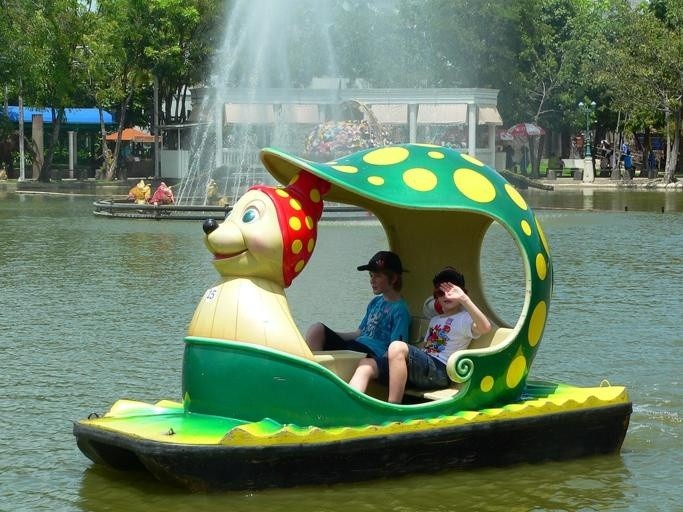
[357,251,402,271]
[433,267,465,296]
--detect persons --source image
[306,251,412,358]
[348,269,492,403]
[600,149,636,177]
[548,153,565,176]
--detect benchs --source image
[547,168,583,180]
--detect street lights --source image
[577,99,599,184]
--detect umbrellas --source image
[506,122,545,171]
[494,128,514,141]
[106,128,160,143]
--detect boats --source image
[67,138,636,478]
